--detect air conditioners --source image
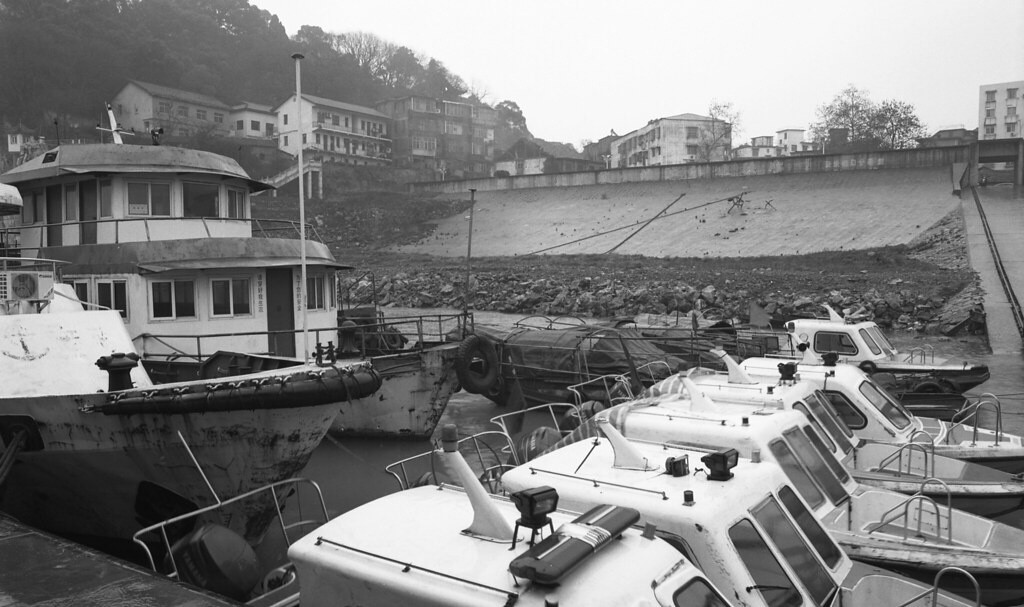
[0,270,54,301]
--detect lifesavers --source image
[385,329,401,348]
[455,335,499,394]
[859,360,876,371]
[488,376,506,398]
[910,376,955,393]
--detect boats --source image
[386,300,1024,607]
[133,420,736,607]
[0,180,385,573]
[0,51,474,442]
